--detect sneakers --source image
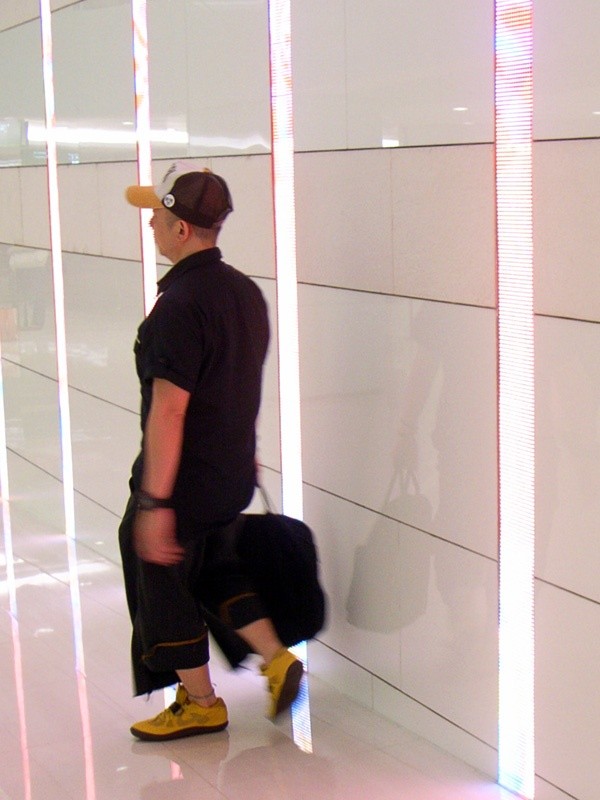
[130,685,228,740]
[261,650,304,716]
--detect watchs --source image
[134,486,174,510]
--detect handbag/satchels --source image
[211,483,326,668]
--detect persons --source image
[116,164,305,744]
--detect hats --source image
[126,163,233,228]
